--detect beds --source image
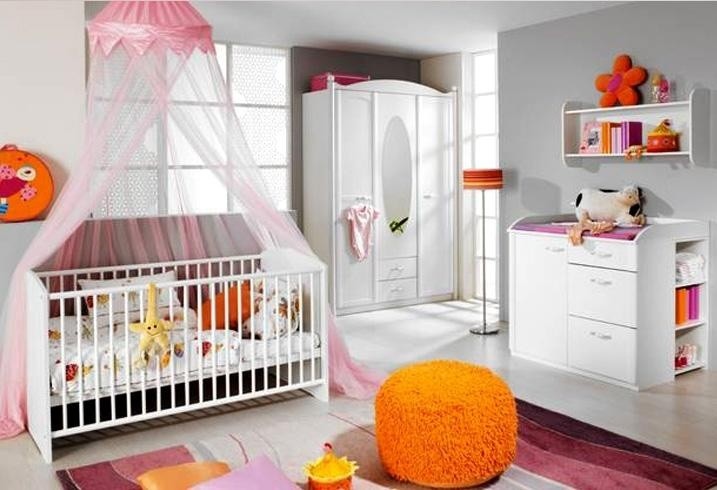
[22,247,331,466]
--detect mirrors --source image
[380,113,412,237]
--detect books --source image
[601,121,644,154]
[676,286,704,325]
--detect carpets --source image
[55,395,716,489]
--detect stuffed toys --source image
[573,183,647,229]
[0,142,56,223]
[594,53,648,106]
[661,78,671,102]
[676,344,688,369]
[302,439,361,490]
[128,279,176,373]
[651,69,661,103]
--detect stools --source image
[373,359,518,490]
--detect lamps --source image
[462,166,504,335]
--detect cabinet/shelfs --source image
[560,87,711,168]
[300,74,461,317]
[506,212,709,392]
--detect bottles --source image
[650,71,669,103]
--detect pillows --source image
[76,270,254,329]
